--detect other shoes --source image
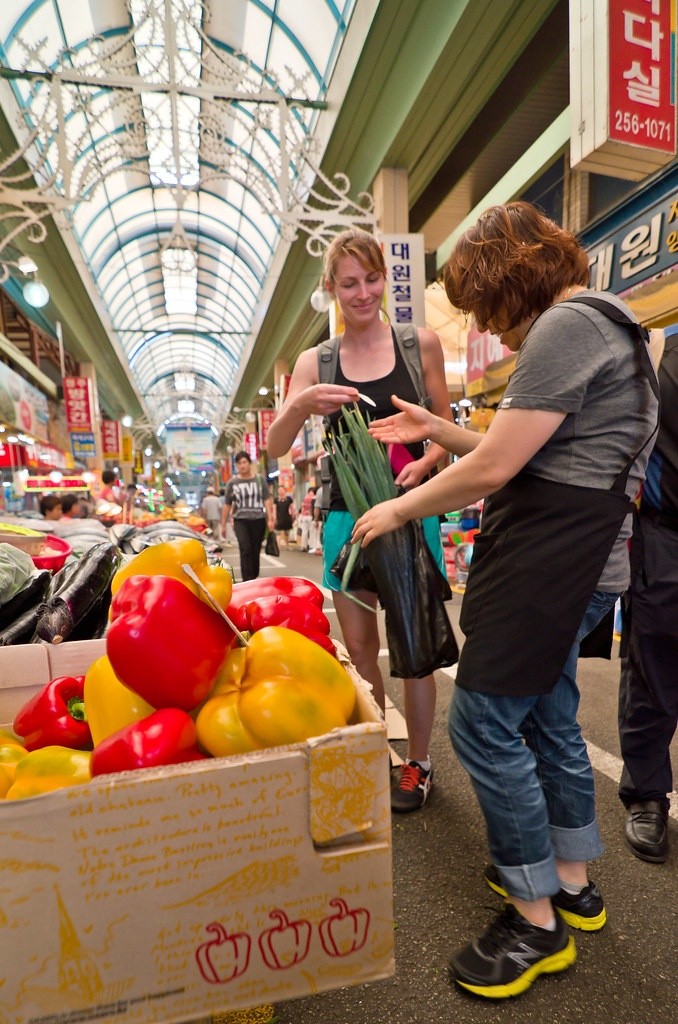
[301,545,323,555]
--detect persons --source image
[221,451,275,582]
[268,232,454,814]
[200,486,331,556]
[40,471,188,532]
[350,200,658,996]
[619,332,678,862]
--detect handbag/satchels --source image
[330,484,460,679]
[265,531,280,557]
[297,528,310,539]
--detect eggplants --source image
[35,541,124,645]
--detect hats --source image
[207,486,215,493]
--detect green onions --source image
[324,398,399,613]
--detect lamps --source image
[311,281,329,312]
[18,256,50,307]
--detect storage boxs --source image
[0,638,395,1024]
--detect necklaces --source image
[563,287,571,301]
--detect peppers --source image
[0,575,358,803]
[109,538,233,618]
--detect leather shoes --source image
[625,799,669,862]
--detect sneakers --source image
[484,862,607,930]
[448,900,576,998]
[391,761,434,812]
